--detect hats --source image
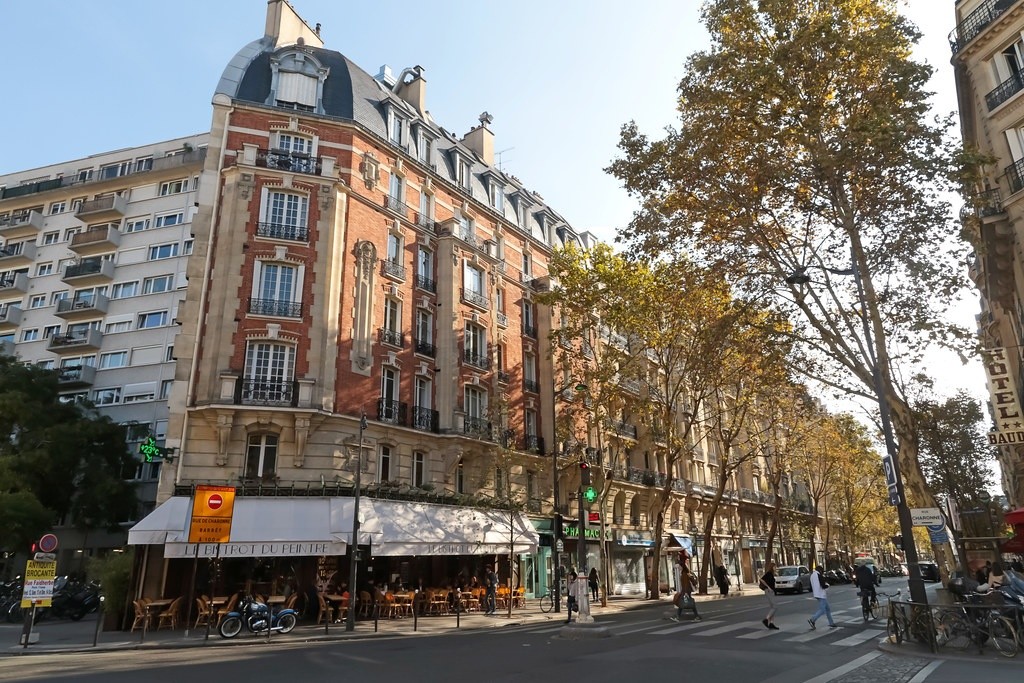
[569,572,576,581]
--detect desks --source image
[267,595,286,610]
[325,594,347,623]
[460,592,472,614]
[206,597,228,629]
[145,599,174,633]
[377,594,410,619]
[425,594,443,616]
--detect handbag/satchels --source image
[589,581,592,587]
[673,593,694,609]
[572,604,578,612]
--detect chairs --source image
[359,586,526,620]
[316,593,334,625]
[131,595,185,633]
[194,593,298,629]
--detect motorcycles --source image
[218,588,300,640]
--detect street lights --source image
[345,409,368,635]
[786,246,938,638]
[554,379,588,613]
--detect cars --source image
[773,565,813,595]
[917,560,942,583]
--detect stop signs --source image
[208,493,223,510]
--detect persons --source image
[855,563,879,608]
[758,562,779,630]
[716,565,731,595]
[566,568,600,612]
[670,562,703,623]
[329,563,500,623]
[808,566,838,628]
[979,557,1019,587]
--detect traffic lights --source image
[580,462,591,486]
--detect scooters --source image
[948,562,1024,650]
[817,553,909,590]
[0,567,105,625]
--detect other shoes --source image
[670,617,679,623]
[807,619,816,629]
[769,623,779,629]
[690,617,702,623]
[763,619,768,628]
[829,624,837,629]
[486,610,497,615]
[565,620,571,623]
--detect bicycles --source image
[940,587,1019,659]
[540,584,571,613]
[873,590,944,646]
[856,581,881,622]
